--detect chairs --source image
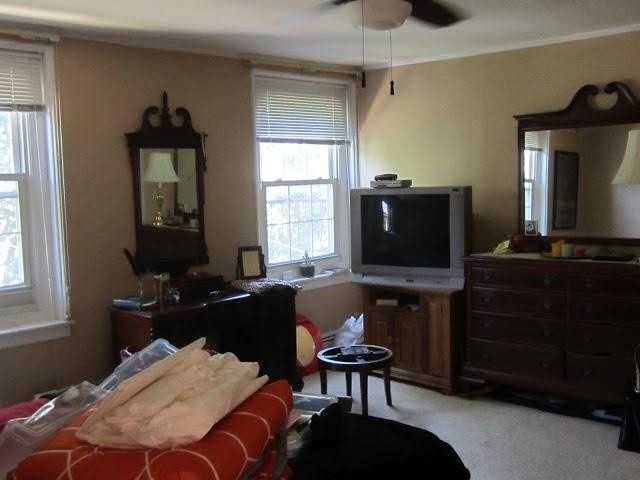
[281,385,355,466]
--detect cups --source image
[551,243,564,256]
[576,248,585,256]
[561,244,574,257]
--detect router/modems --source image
[375,174,397,180]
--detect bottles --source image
[189,207,199,229]
[153,274,171,303]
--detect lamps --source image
[611,128,640,185]
[342,0,411,30]
[140,151,180,226]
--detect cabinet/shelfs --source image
[458,252,640,426]
[356,283,464,394]
[103,279,305,393]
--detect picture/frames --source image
[553,150,579,231]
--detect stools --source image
[317,343,394,415]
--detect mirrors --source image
[239,244,264,279]
[512,81,640,245]
[122,90,210,268]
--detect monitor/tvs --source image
[350,185,472,290]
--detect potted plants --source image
[301,251,315,278]
